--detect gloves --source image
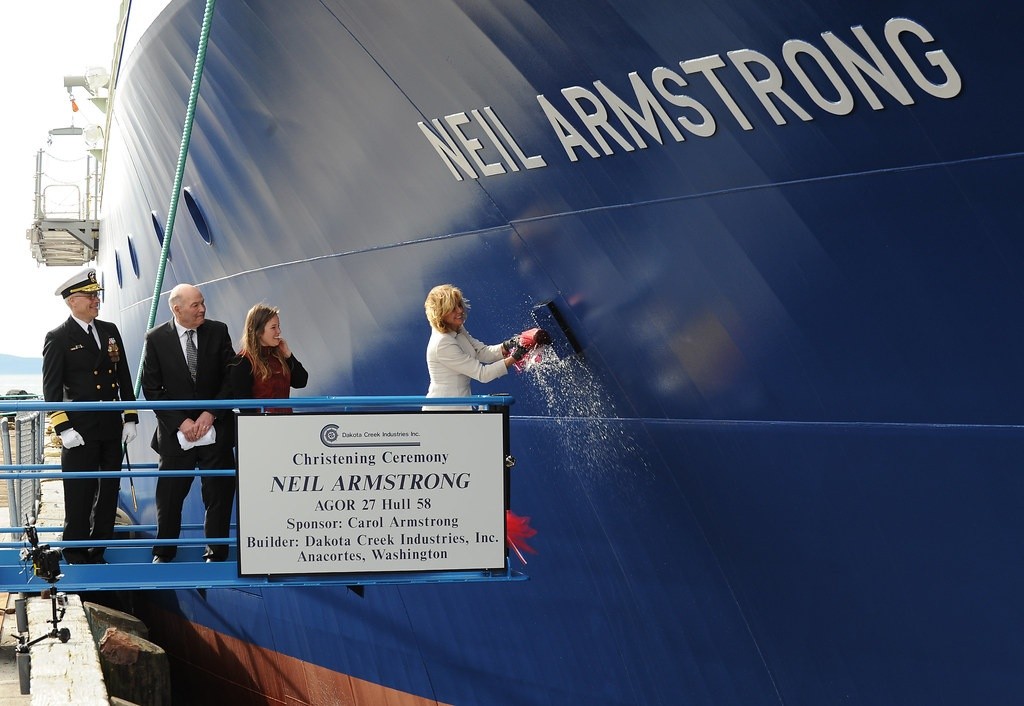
[502,336,529,361]
[60,426,86,449]
[121,420,138,445]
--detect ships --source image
[25,2,1022,706]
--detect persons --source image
[422,284,537,411]
[42,268,140,564]
[230,303,309,413]
[140,284,236,563]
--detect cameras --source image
[34,547,63,582]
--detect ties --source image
[183,330,199,388]
[86,321,101,351]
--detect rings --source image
[202,425,209,430]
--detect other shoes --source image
[151,555,168,565]
[204,557,222,563]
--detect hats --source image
[54,267,105,304]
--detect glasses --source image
[72,290,100,300]
